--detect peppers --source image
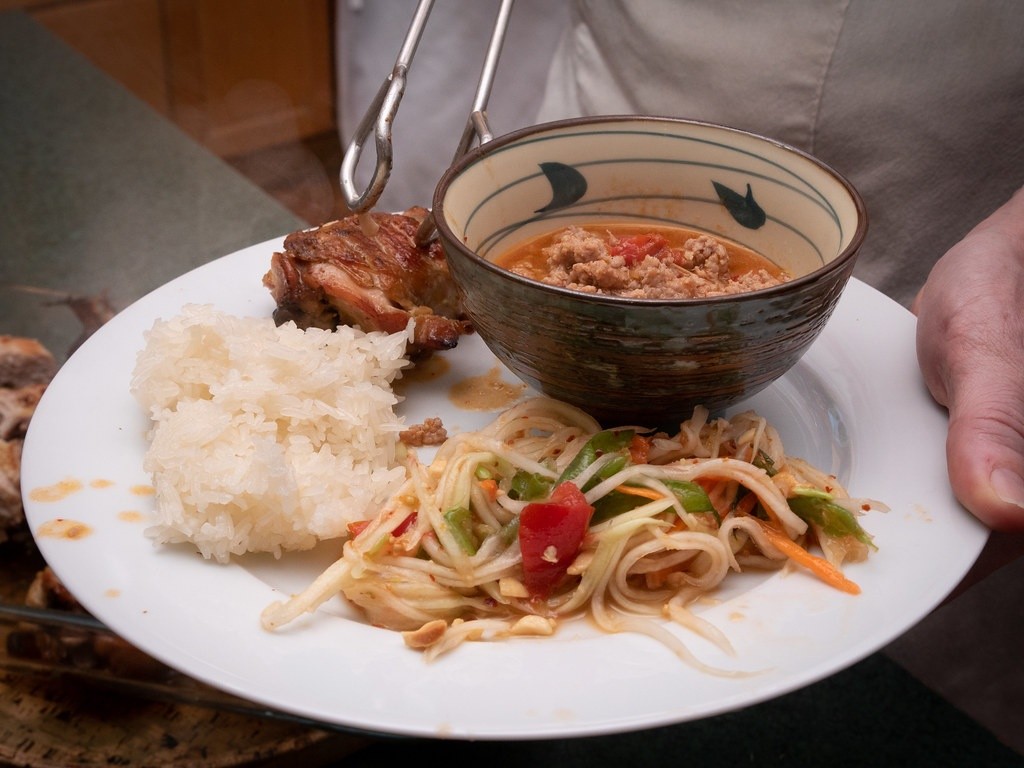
[444,425,877,599]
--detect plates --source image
[19,211,991,740]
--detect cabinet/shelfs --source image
[27,0,330,158]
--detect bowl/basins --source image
[434,115,868,413]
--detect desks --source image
[0,0,1023,768]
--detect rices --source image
[131,300,416,566]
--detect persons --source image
[534,0,1024,754]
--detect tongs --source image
[338,0,512,246]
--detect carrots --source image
[738,507,860,596]
